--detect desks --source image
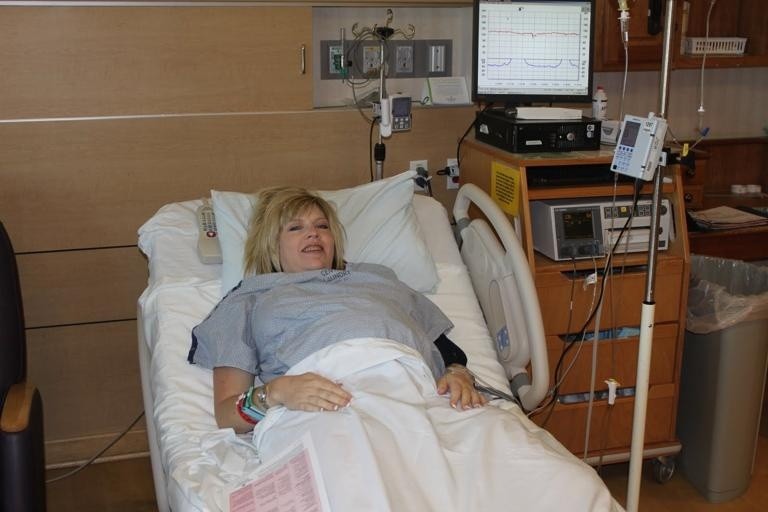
[682,209,768,264]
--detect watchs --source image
[257,383,272,409]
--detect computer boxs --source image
[475,109,601,153]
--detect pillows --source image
[204,172,440,295]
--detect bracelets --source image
[237,388,265,426]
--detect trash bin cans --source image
[671,255,768,503]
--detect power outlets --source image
[329,47,349,75]
[445,159,460,191]
[395,47,414,74]
[410,159,430,194]
[363,48,384,74]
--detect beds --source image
[134,191,628,509]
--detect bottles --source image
[593,86,607,122]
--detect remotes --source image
[197,204,222,264]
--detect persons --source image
[186,187,490,433]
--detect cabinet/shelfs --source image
[456,129,693,451]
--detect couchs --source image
[1,219,48,511]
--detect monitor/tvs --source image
[472,0,595,102]
